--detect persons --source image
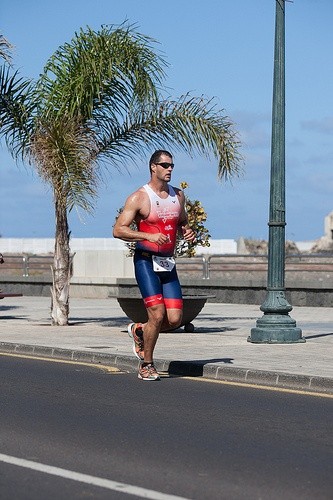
[113,149,196,381]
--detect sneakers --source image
[137,360,160,381]
[128,322,144,360]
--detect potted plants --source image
[110,183,218,331]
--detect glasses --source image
[153,162,174,169]
